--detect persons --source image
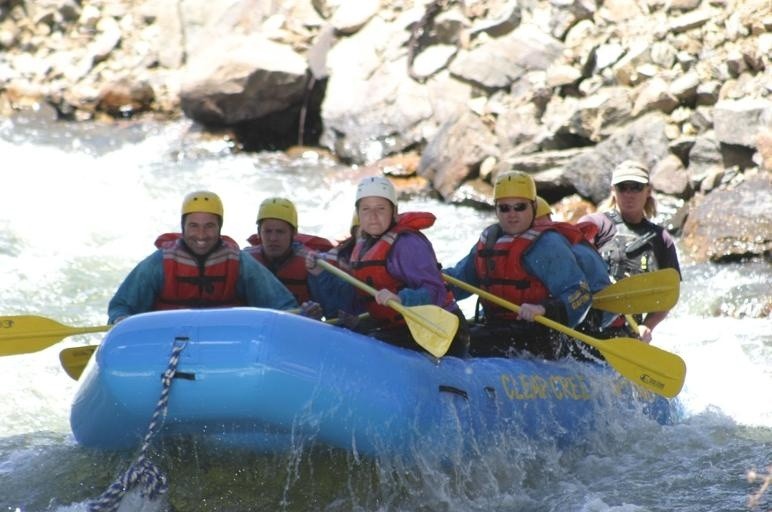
[440,169,593,358]
[533,193,636,366]
[575,159,682,343]
[242,196,359,330]
[304,174,471,361]
[107,190,323,325]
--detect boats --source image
[70,308,683,472]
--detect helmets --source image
[256,197,298,230]
[534,196,552,219]
[494,172,536,201]
[181,192,223,231]
[354,177,398,208]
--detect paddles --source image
[312,254,460,361]
[437,263,686,400]
[58,345,97,380]
[0,315,114,357]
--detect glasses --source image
[498,204,527,212]
[614,183,645,191]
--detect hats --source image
[612,167,649,185]
